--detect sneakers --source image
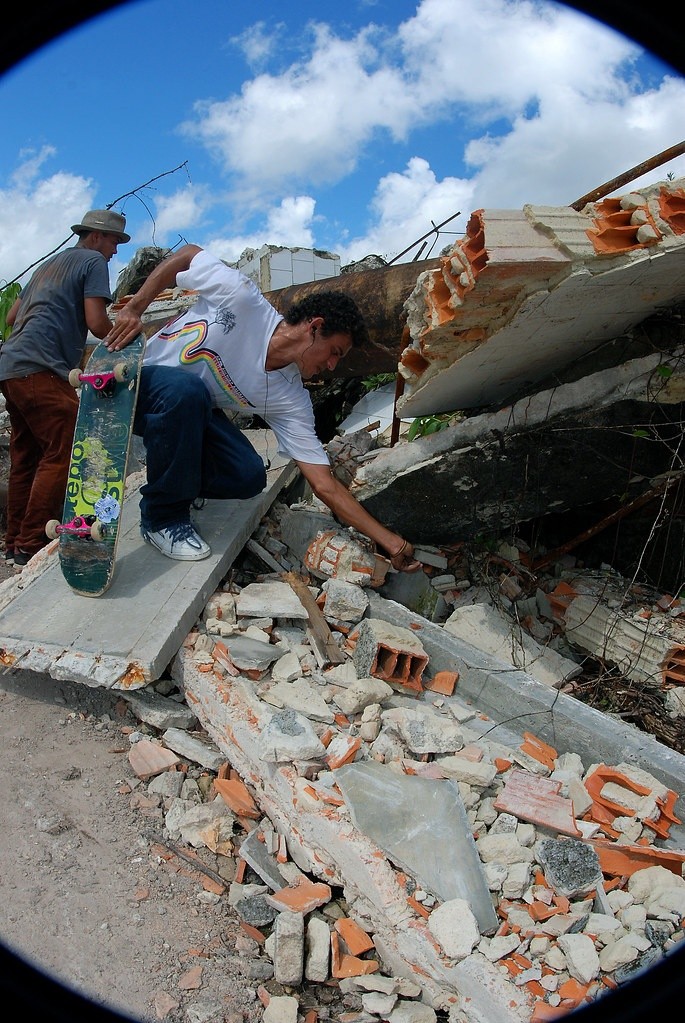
[140,523,211,561]
[192,497,209,510]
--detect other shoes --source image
[13,546,33,570]
[5,544,15,564]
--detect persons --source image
[0,209,131,570]
[104,244,424,573]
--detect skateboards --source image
[45,332,150,599]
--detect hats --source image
[71,210,131,243]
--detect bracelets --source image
[390,540,407,558]
